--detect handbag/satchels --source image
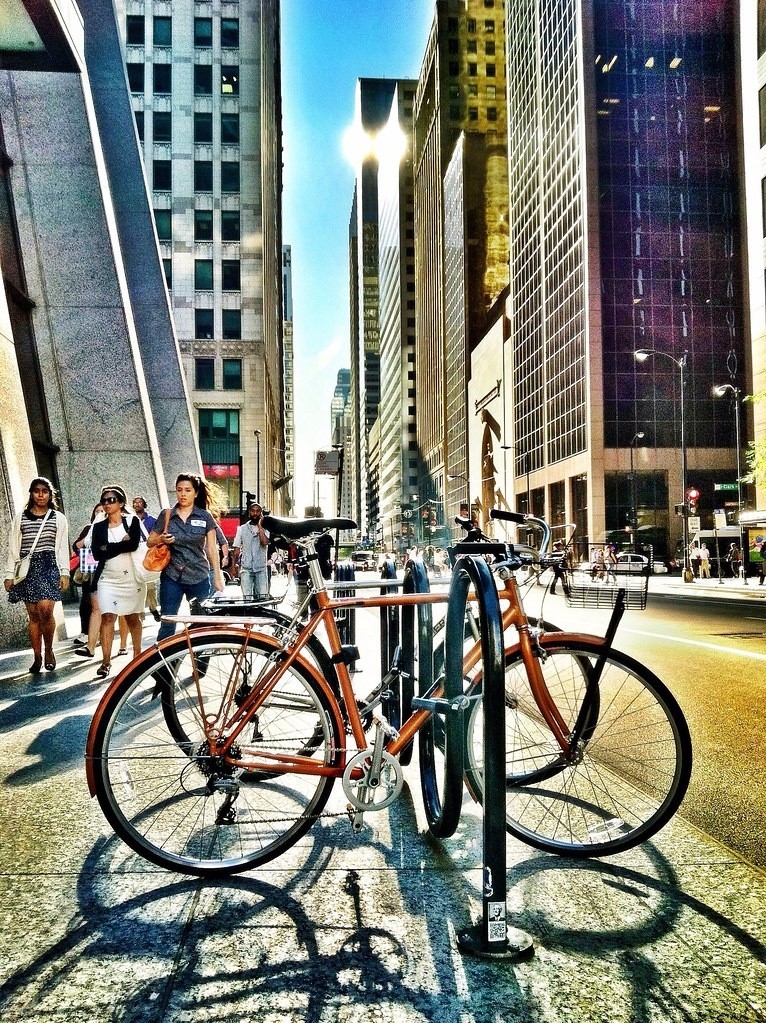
[69,552,80,571]
[73,567,90,584]
[80,548,100,572]
[737,552,743,559]
[12,554,32,585]
[142,508,171,571]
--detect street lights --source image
[450,476,470,521]
[501,446,532,546]
[373,520,383,546]
[379,515,393,553]
[416,496,431,562]
[396,500,410,548]
[635,348,694,583]
[254,429,262,504]
[711,384,749,586]
[630,432,645,553]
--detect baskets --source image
[559,543,653,609]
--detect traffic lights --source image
[687,489,700,515]
[246,491,256,505]
[674,504,683,514]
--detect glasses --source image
[100,497,120,505]
[134,501,144,506]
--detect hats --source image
[101,486,126,501]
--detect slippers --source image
[75,647,94,657]
[118,646,127,654]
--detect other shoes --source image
[193,667,205,676]
[149,606,161,621]
[537,583,544,586]
[550,590,556,595]
[73,635,88,645]
[29,665,40,672]
[301,616,308,623]
[97,666,110,675]
[45,657,56,669]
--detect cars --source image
[352,550,377,572]
[579,552,668,576]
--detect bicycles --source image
[83,509,694,878]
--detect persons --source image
[75,484,161,676]
[230,504,270,602]
[409,546,450,577]
[699,543,711,578]
[760,541,766,560]
[727,543,739,577]
[148,472,224,682]
[73,503,105,645]
[204,523,228,595]
[287,532,334,621]
[524,542,617,599]
[4,478,70,674]
[689,543,699,577]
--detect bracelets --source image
[225,557,229,559]
